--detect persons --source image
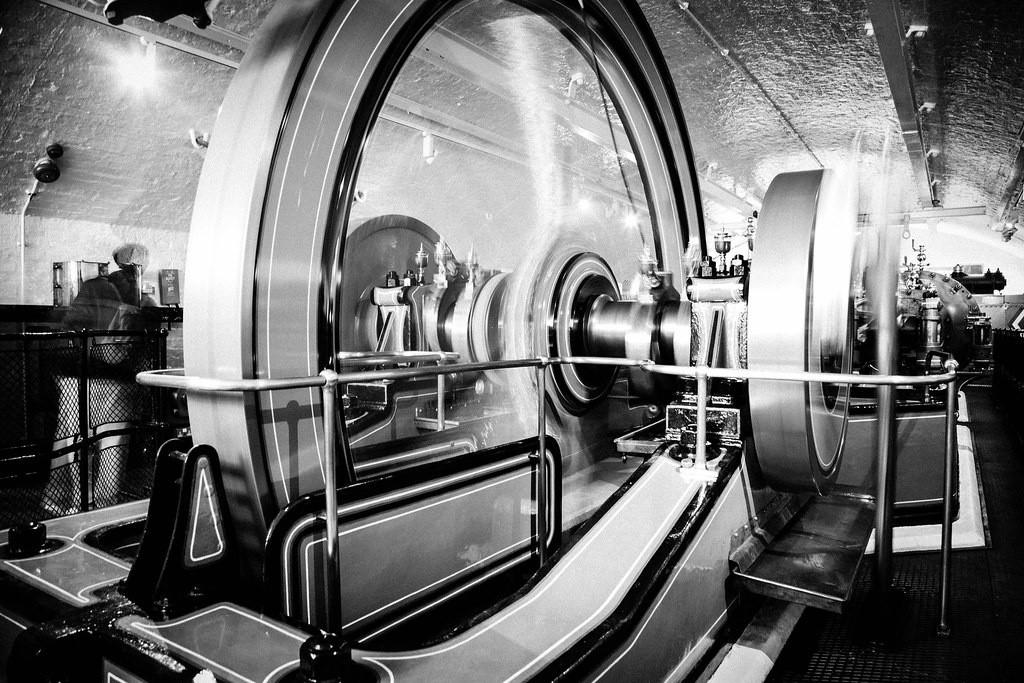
[39,243,158,519]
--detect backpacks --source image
[50,276,151,365]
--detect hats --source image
[112,243,152,268]
